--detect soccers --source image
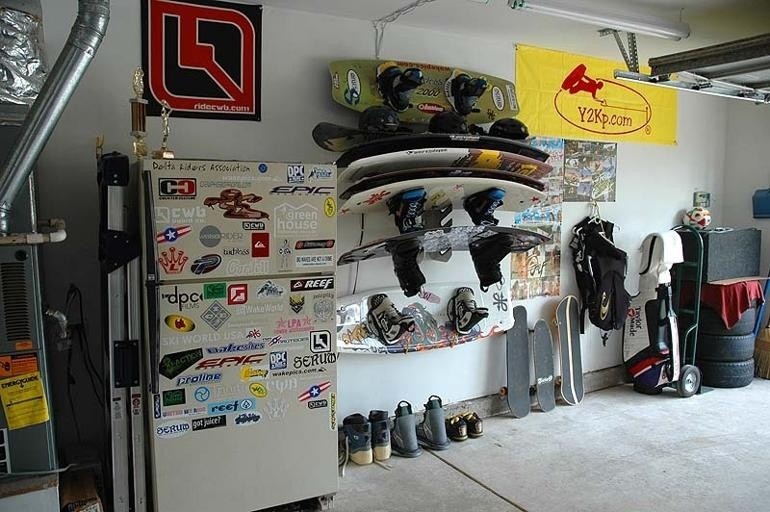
[683,206,711,230]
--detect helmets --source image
[427,110,468,134]
[490,116,530,139]
[359,107,401,134]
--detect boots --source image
[451,71,487,114]
[468,235,513,287]
[369,409,394,461]
[385,238,426,295]
[342,413,374,465]
[417,394,450,452]
[378,60,425,111]
[392,399,421,457]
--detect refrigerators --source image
[128,158,340,511]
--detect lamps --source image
[508,0,691,42]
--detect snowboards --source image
[335,284,517,354]
[313,106,553,231]
[336,224,550,296]
[328,59,519,123]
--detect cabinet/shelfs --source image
[670,224,762,394]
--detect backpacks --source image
[589,232,629,330]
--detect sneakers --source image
[453,287,489,336]
[446,413,470,440]
[464,409,484,437]
[462,188,506,227]
[365,294,415,345]
[385,186,431,234]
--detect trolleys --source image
[665,224,704,399]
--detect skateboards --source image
[500,305,531,418]
[555,295,584,406]
[530,318,556,412]
